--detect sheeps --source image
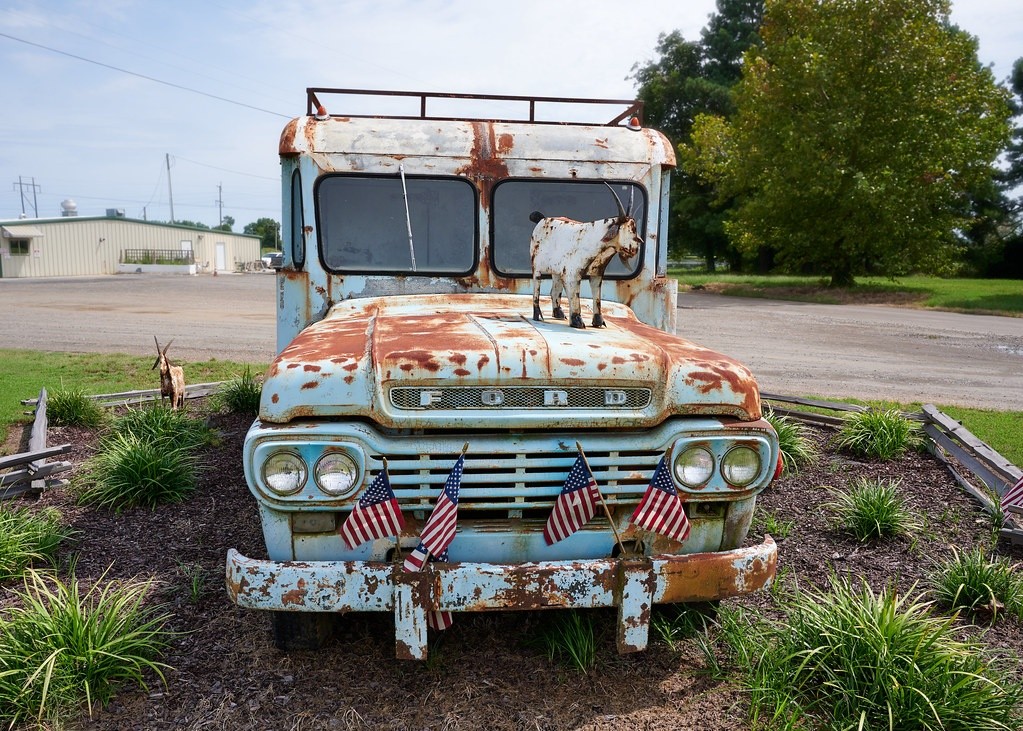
[529,182,645,329]
[154,336,185,409]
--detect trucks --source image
[224,87,783,661]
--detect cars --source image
[260,252,283,272]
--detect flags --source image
[338,467,406,551]
[630,456,691,543]
[420,454,466,558]
[543,452,599,546]
[403,543,454,630]
